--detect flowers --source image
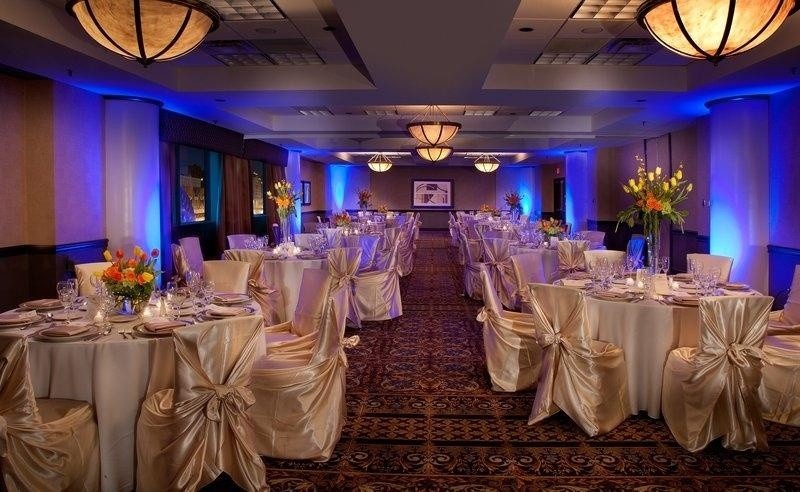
[266,178,299,217]
[614,148,693,245]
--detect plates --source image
[725,282,750,291]
[0,292,257,342]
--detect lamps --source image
[474,153,502,172]
[415,141,453,162]
[635,0,800,68]
[368,153,393,172]
[407,104,462,145]
[67,0,222,68]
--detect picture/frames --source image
[412,179,455,209]
[303,181,312,206]
[299,181,303,207]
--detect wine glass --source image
[469,210,723,296]
[55,212,399,328]
